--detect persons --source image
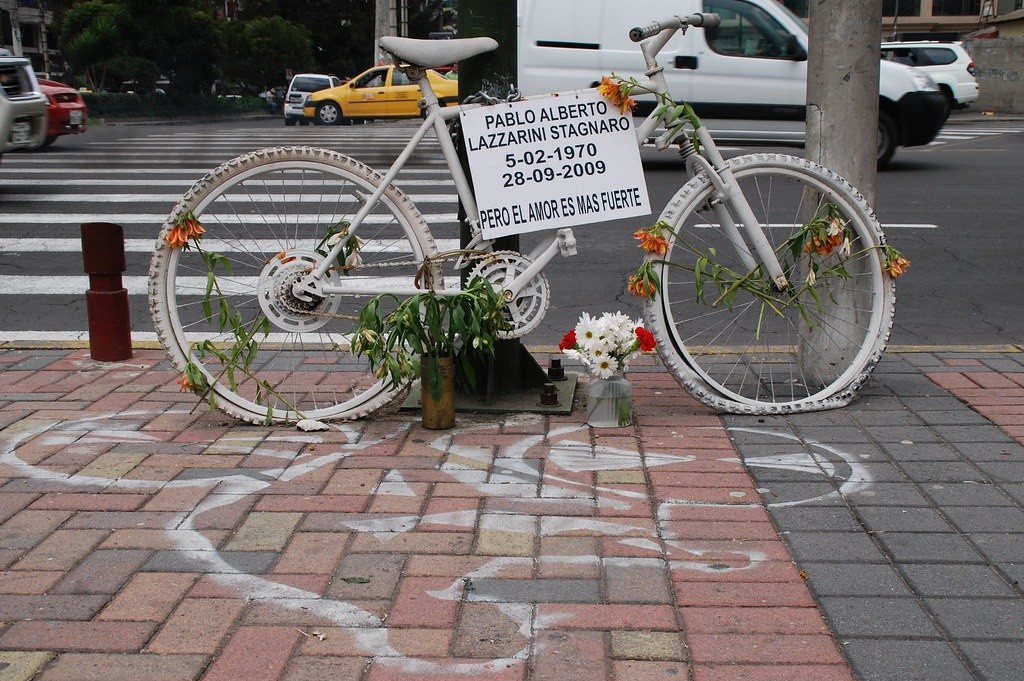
[267,87,276,112]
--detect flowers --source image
[597,72,703,155]
[314,221,365,276]
[188,247,297,417]
[348,276,515,404]
[166,209,253,351]
[625,202,911,341]
[559,310,657,426]
[182,338,308,426]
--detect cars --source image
[302,64,458,126]
[36,76,89,150]
[116,78,286,105]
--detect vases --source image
[586,370,634,428]
[421,352,455,431]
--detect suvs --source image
[875,38,982,111]
[282,73,341,124]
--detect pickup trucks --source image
[0,55,47,153]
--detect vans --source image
[515,0,951,176]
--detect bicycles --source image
[146,16,896,431]
